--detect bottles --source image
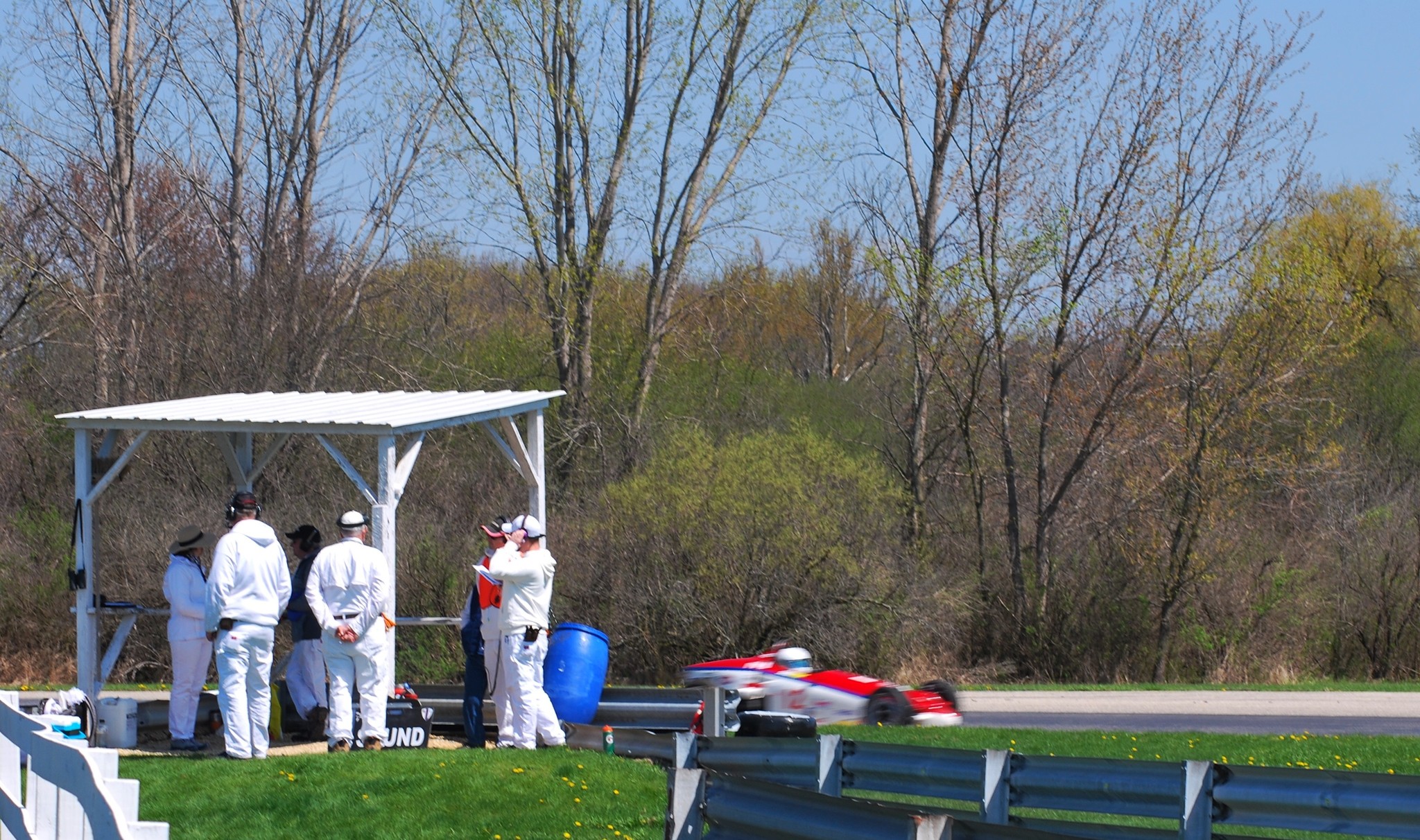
[602,724,614,756]
[96,719,107,748]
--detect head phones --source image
[300,529,319,552]
[226,492,261,521]
[519,515,528,539]
[337,514,370,528]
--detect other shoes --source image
[292,733,327,743]
[307,706,328,737]
[363,737,382,752]
[328,741,350,753]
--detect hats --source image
[286,525,320,542]
[501,515,541,538]
[480,516,513,538]
[227,491,257,511]
[169,525,216,554]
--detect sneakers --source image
[170,739,198,751]
[191,738,208,750]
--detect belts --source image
[333,614,357,621]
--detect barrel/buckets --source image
[543,621,609,724]
[94,696,138,749]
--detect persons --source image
[162,526,213,749]
[461,513,568,750]
[284,524,329,743]
[203,491,292,761]
[305,511,390,753]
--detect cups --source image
[208,709,221,733]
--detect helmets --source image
[776,646,813,673]
